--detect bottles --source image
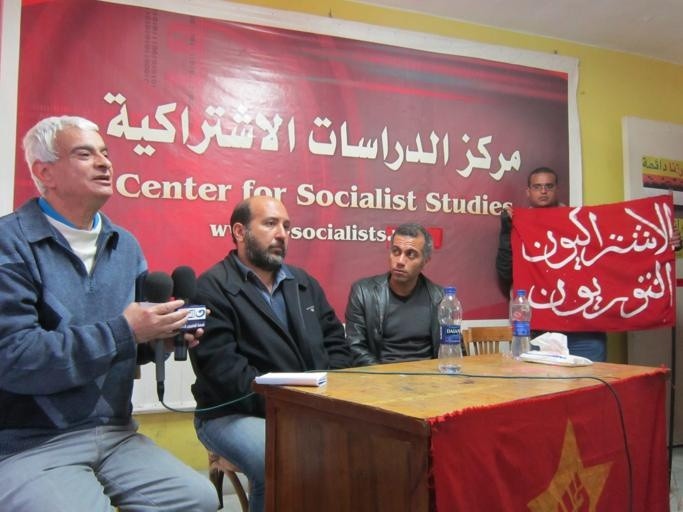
[436,288,464,375]
[508,290,531,358]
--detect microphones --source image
[144,272,174,392]
[170,267,206,362]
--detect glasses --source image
[528,183,558,192]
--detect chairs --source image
[207,451,249,511]
[461,327,513,355]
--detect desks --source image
[251,354,671,512]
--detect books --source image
[254,371,328,387]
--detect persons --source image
[494,165,681,363]
[344,222,467,368]
[190,194,357,512]
[0,114,221,512]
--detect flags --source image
[510,191,677,333]
